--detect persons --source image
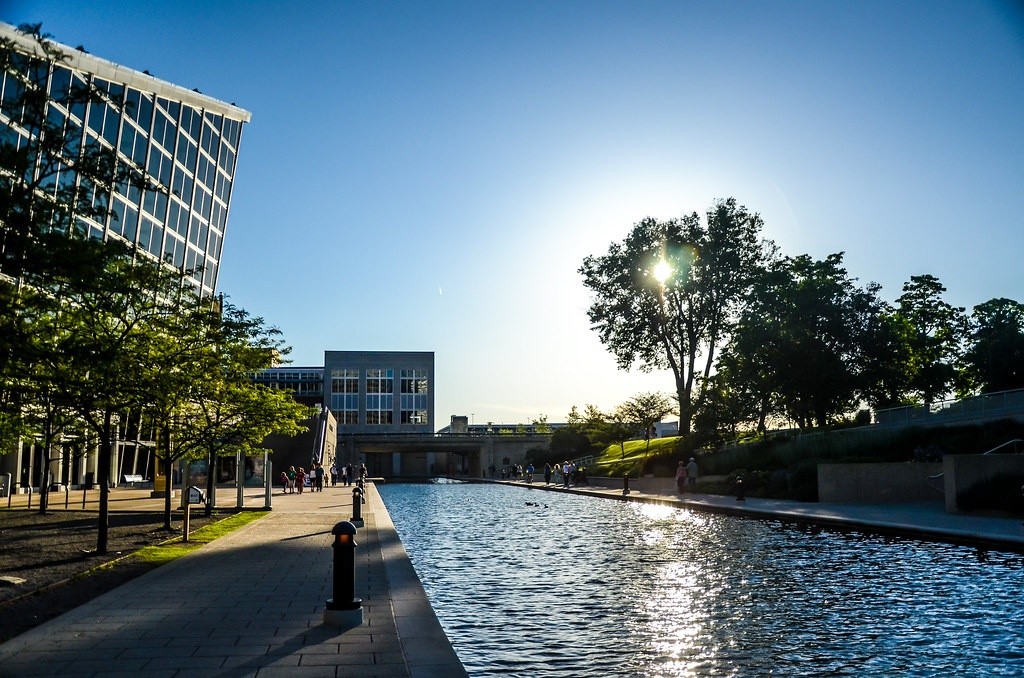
[674,457,698,494]
[279,463,329,495]
[488,463,535,483]
[330,462,368,488]
[544,460,577,487]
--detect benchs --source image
[124,475,151,488]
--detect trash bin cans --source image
[85,472,95,489]
